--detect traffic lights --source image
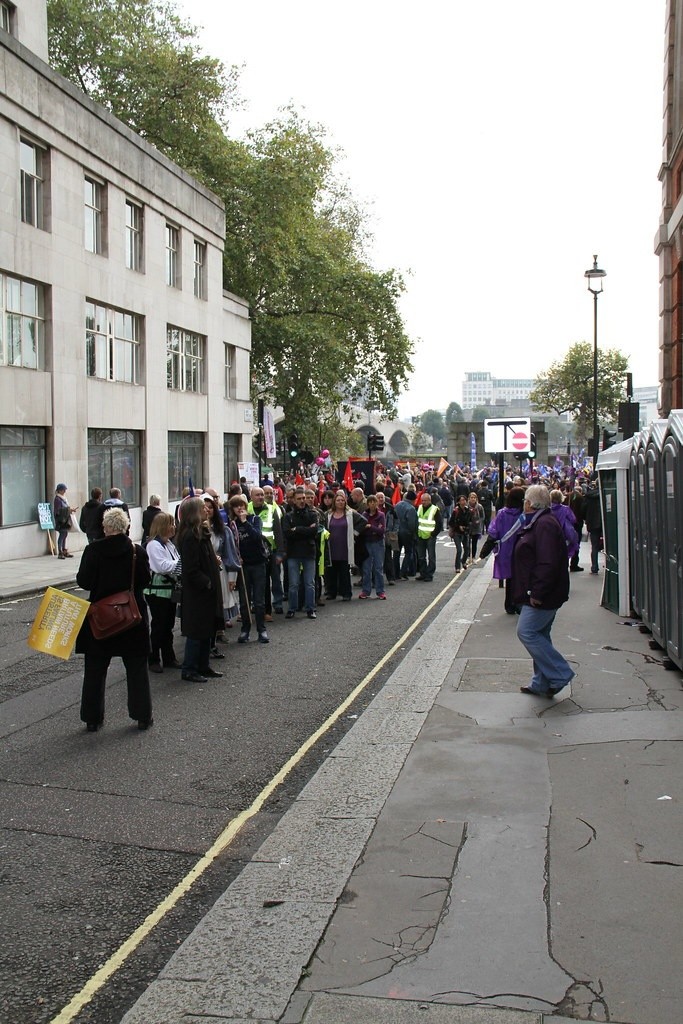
[513,452,526,461]
[375,435,384,450]
[602,428,617,451]
[526,433,537,459]
[289,434,299,459]
[367,434,375,451]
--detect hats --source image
[56,484,67,491]
[406,491,416,499]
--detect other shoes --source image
[352,557,477,587]
[87,720,104,732]
[200,667,223,676]
[546,680,569,697]
[570,564,584,571]
[170,655,184,668]
[211,578,352,658]
[138,718,153,730]
[149,663,163,673]
[183,671,207,682]
[592,570,598,575]
[519,685,541,696]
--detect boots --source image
[58,549,74,559]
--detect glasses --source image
[212,496,219,499]
[521,498,527,502]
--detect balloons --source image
[316,457,325,466]
[321,449,329,458]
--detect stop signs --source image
[511,432,529,449]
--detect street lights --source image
[583,254,607,476]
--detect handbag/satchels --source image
[389,532,400,551]
[87,589,142,641]
[55,517,71,532]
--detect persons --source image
[75,507,154,730]
[98,487,131,536]
[480,488,525,614]
[54,484,76,559]
[80,488,106,542]
[550,490,579,559]
[143,474,442,683]
[510,486,575,698]
[287,462,605,574]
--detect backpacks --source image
[449,506,472,538]
[252,515,272,562]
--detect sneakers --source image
[377,592,386,600]
[359,591,371,599]
